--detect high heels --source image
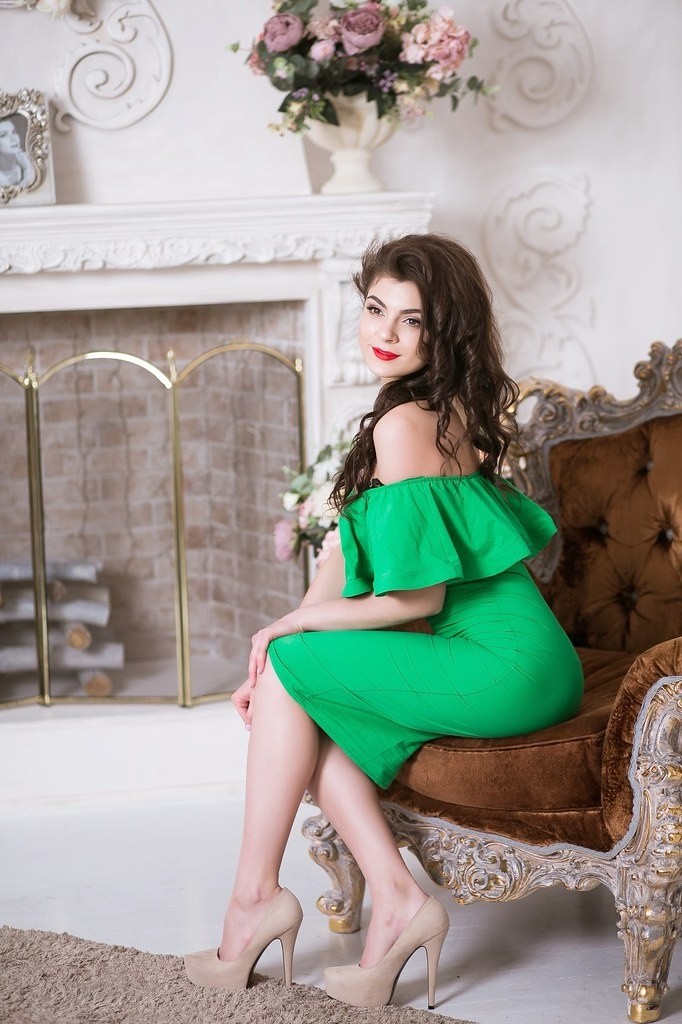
[323,894,450,1010]
[183,887,303,989]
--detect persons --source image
[183,226,584,1014]
[0,118,33,188]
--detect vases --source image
[287,87,403,195]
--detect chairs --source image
[275,334,682,1024]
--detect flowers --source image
[230,0,502,139]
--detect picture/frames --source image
[0,86,57,208]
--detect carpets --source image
[0,925,486,1024]
